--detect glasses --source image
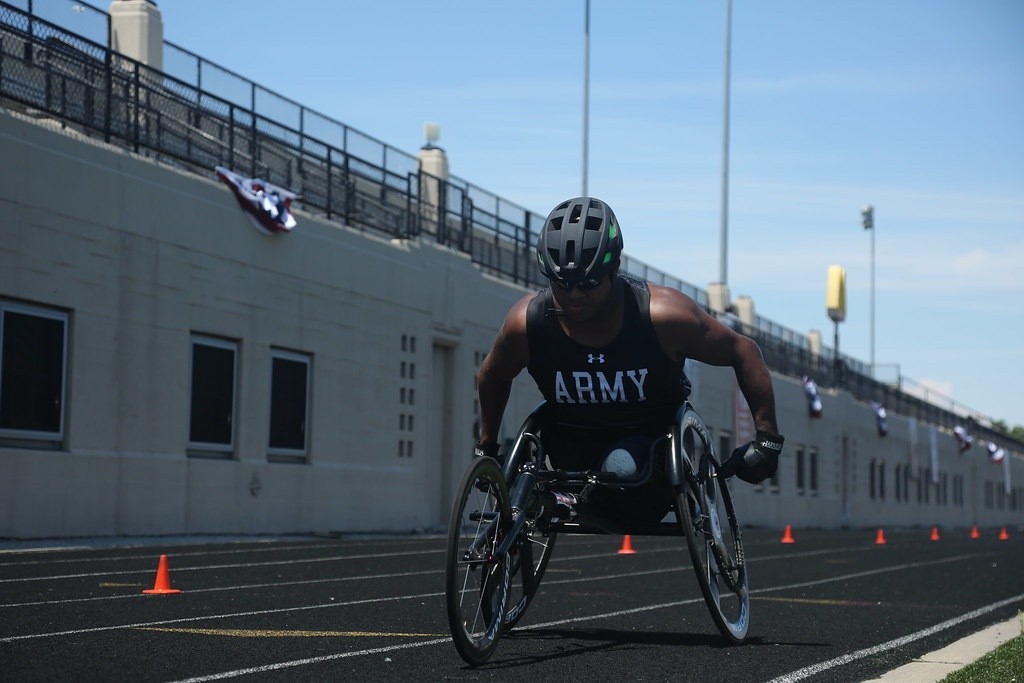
[548,272,605,291]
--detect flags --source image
[862,210,873,229]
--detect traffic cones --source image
[141,554,180,595]
[616,534,637,555]
[999,528,1010,540]
[971,526,981,539]
[780,524,797,546]
[931,528,941,540]
[875,529,888,545]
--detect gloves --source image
[732,429,785,485]
[470,440,506,492]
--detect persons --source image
[471,197,786,538]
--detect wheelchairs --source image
[445,397,756,665]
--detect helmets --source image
[536,197,623,280]
